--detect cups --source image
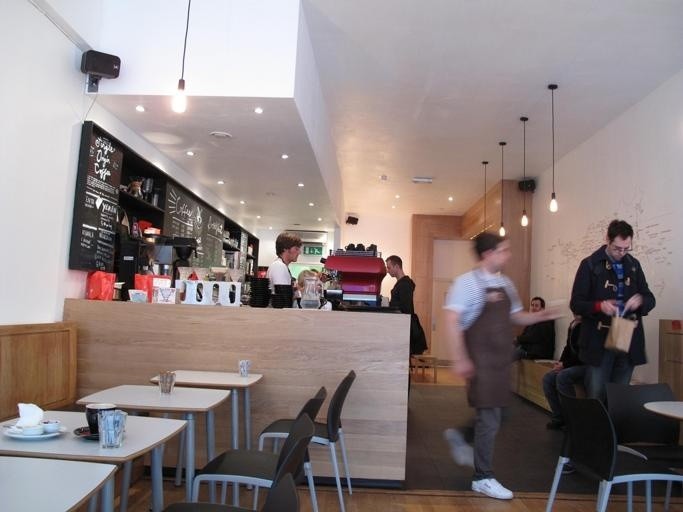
[150,193,158,206]
[237,359,250,378]
[159,369,175,397]
[85,401,117,436]
[344,242,377,251]
[221,258,226,266]
[380,296,389,307]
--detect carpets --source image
[404,387,682,498]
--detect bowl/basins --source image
[41,419,59,432]
[21,425,42,435]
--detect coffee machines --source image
[138,236,199,282]
[320,254,387,306]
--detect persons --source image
[559,219,655,476]
[265,232,302,295]
[542,315,581,430]
[511,297,556,361]
[444,231,566,501]
[385,255,416,314]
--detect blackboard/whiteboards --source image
[239,230,248,271]
[163,178,225,268]
[69,121,123,273]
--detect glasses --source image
[612,242,633,253]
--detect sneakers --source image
[471,477,514,500]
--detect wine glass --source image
[99,409,126,448]
[141,177,153,202]
[159,288,173,302]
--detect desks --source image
[0,383,150,511]
[642,399,683,446]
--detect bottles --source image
[204,273,215,281]
[128,174,145,200]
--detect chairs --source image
[605,383,683,511]
[149,367,358,512]
[542,385,683,512]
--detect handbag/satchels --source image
[603,316,637,354]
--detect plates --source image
[73,425,104,441]
[248,276,292,309]
[1,419,67,440]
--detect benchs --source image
[509,353,562,416]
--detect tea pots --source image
[300,277,324,308]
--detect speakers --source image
[347,216,358,224]
[518,180,536,191]
[81,50,121,79]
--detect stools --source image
[409,354,440,384]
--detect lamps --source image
[496,82,558,239]
[167,0,191,119]
